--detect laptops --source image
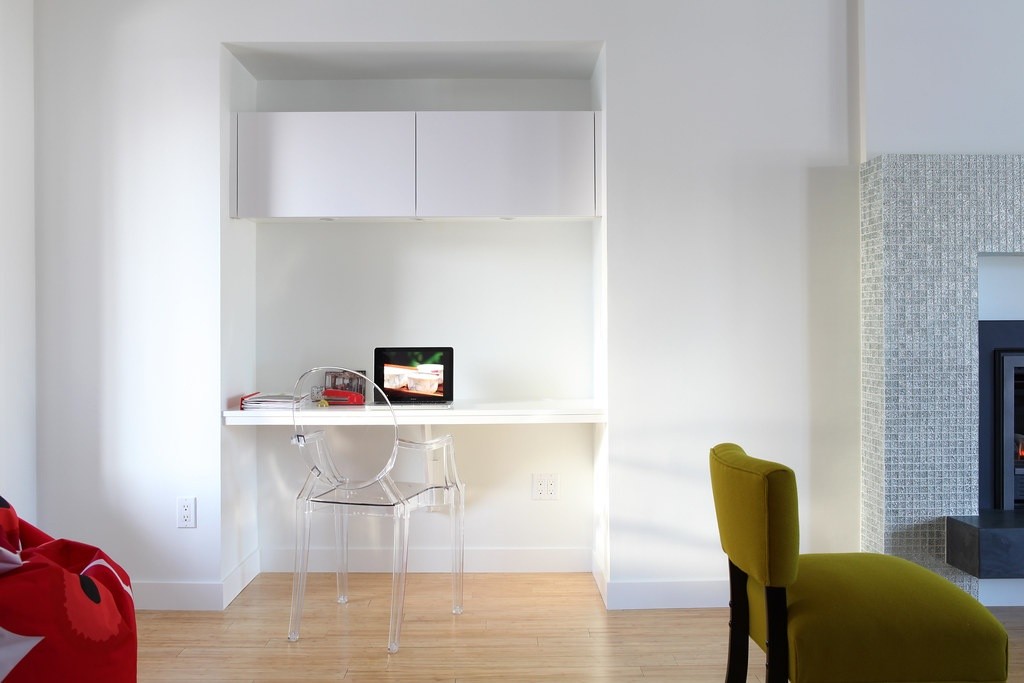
[365,347,455,409]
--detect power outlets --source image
[176,497,196,528]
[531,474,560,501]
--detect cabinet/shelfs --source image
[231,112,600,220]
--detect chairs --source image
[286,366,466,654]
[709,443,1010,683]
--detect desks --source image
[224,398,606,425]
